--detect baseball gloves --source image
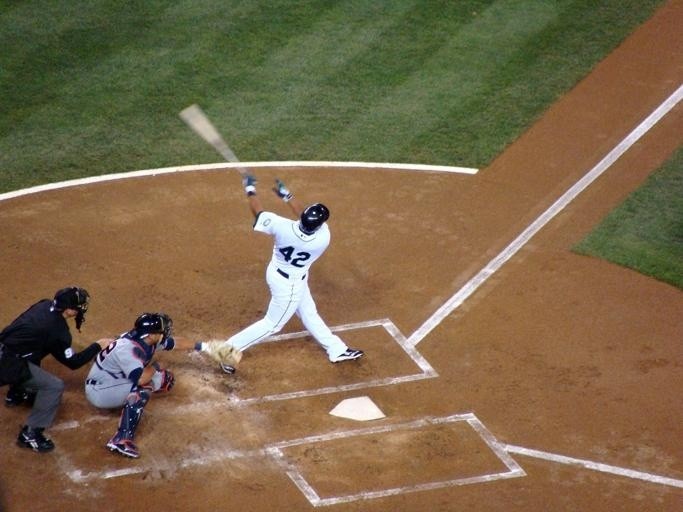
[203,340,242,368]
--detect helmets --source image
[134,313,172,335]
[299,202,329,232]
[53,285,88,310]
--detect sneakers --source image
[106,430,140,457]
[329,348,364,363]
[16,426,55,452]
[218,360,235,375]
[5,389,36,407]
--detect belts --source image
[276,268,306,281]
[84,379,101,385]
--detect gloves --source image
[272,178,294,204]
[241,172,257,196]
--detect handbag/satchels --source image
[0,356,33,388]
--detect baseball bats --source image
[180,104,250,176]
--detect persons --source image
[85,313,176,458]
[0,287,116,452]
[213,176,364,375]
